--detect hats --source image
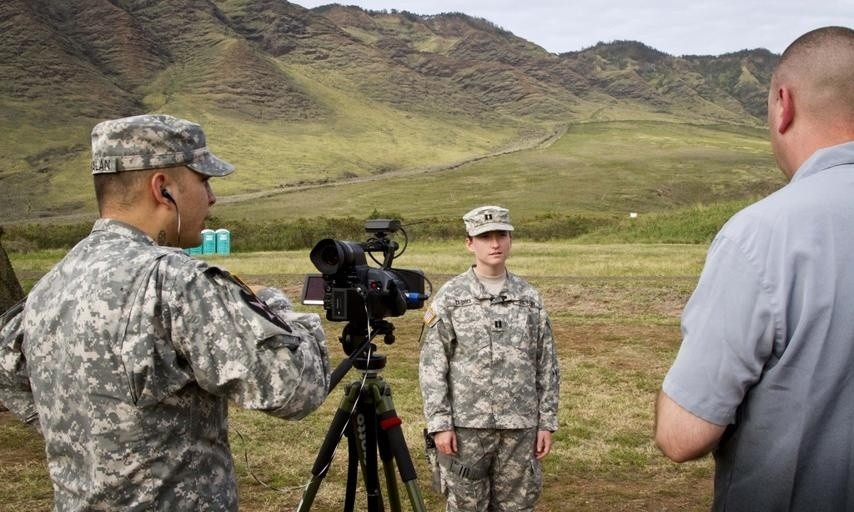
[92,114,236,178]
[462,206,515,238]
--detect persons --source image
[652,27,854,511]
[2,112,332,511]
[419,204,559,512]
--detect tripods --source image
[296,322,428,512]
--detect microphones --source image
[501,295,507,301]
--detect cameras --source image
[301,219,426,321]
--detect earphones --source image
[161,188,178,206]
